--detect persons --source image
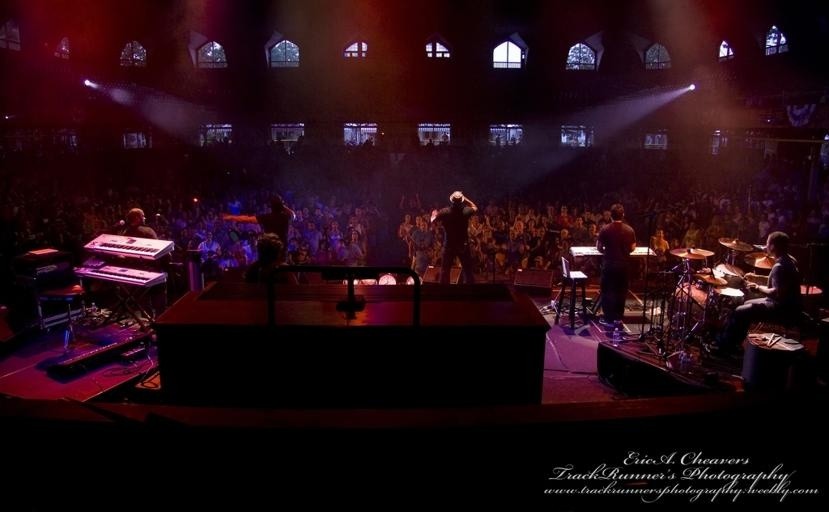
[0,123,828,366]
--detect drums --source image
[667,282,708,332]
[746,273,769,299]
[718,264,744,286]
[707,287,745,329]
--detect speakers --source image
[513,267,553,296]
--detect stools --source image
[555,270,589,329]
[38,281,86,342]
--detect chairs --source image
[755,282,816,338]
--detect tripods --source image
[636,258,704,366]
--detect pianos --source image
[570,244,657,257]
[84,234,175,264]
[72,261,170,289]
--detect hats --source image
[449,190,465,204]
[267,194,285,208]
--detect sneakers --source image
[598,316,615,328]
[613,318,623,324]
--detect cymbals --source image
[718,237,754,252]
[744,251,795,270]
[670,248,715,259]
[801,285,823,296]
[691,273,727,285]
